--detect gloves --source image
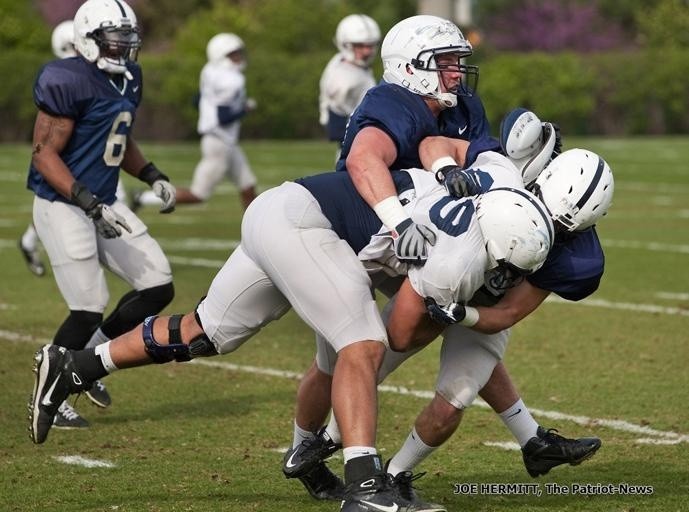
[89,203,132,239]
[435,165,481,200]
[390,218,436,267]
[423,294,466,329]
[152,177,177,214]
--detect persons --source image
[23,132,557,510]
[24,0,178,430]
[19,20,79,277]
[283,146,614,512]
[126,32,259,209]
[281,14,602,501]
[312,12,382,168]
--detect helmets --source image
[474,147,614,291]
[207,33,248,71]
[52,0,142,75]
[381,15,479,109]
[335,13,382,68]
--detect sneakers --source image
[280,424,447,512]
[520,426,601,478]
[26,344,110,444]
[19,239,46,276]
